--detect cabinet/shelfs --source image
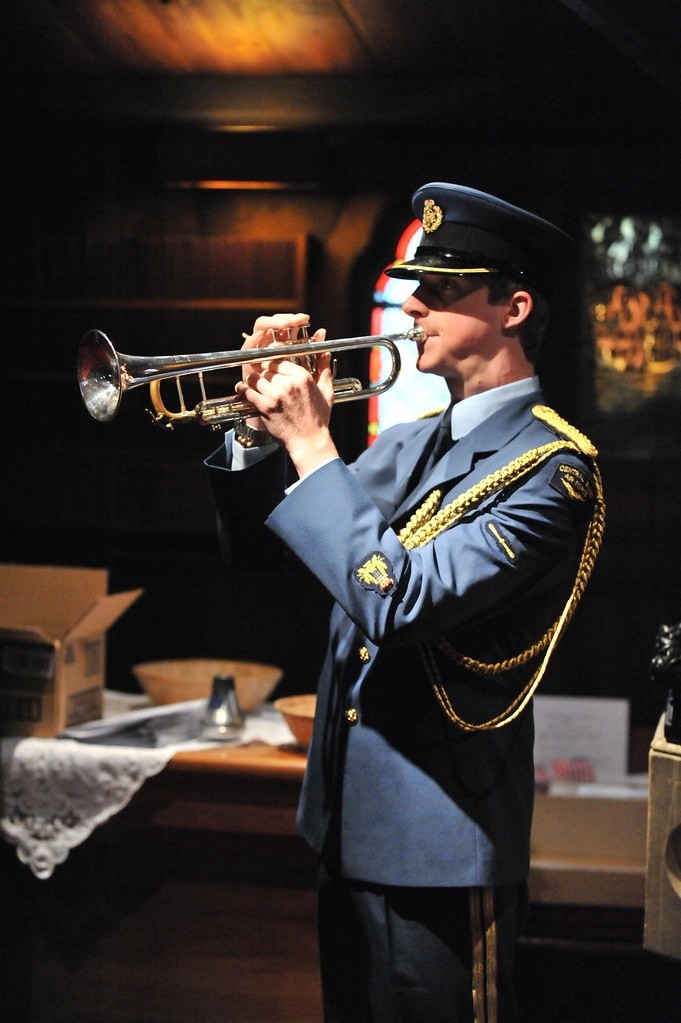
[1,690,323,1023]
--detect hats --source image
[384,182,576,297]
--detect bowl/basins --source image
[274,695,317,752]
[133,660,282,712]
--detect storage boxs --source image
[0,564,144,741]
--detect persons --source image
[230,183,607,1022]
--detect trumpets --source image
[73,327,427,443]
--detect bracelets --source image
[232,418,275,446]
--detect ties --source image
[410,414,452,486]
